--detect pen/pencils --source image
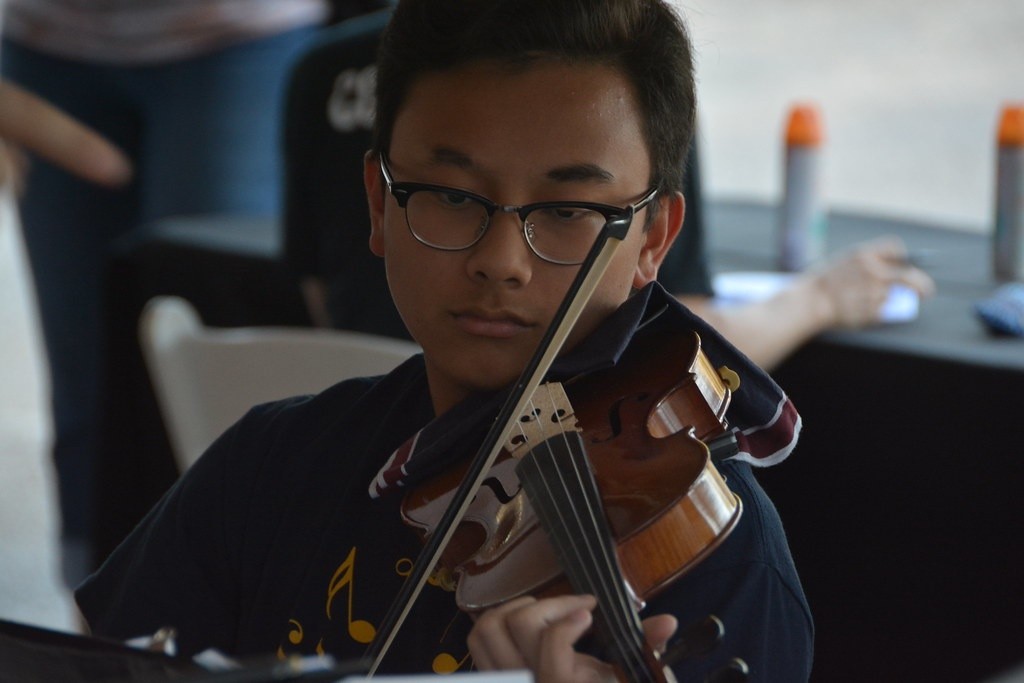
[884,250,951,268]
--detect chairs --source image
[143,291,421,467]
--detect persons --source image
[0,0,933,683]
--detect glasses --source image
[378,150,663,265]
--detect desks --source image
[701,203,1024,683]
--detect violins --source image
[400,322,756,682]
